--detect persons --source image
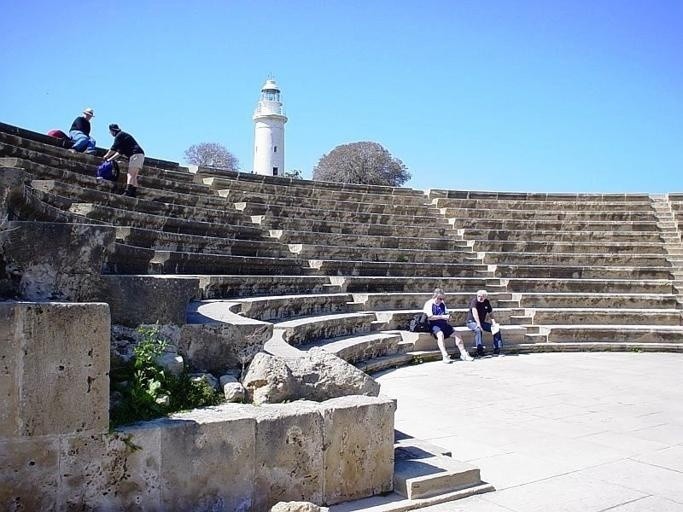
[421,286,473,364]
[68,107,97,155]
[466,289,505,358]
[102,124,145,198]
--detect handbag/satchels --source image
[410,314,429,332]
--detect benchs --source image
[0,121,683,372]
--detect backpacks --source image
[96,159,120,179]
[49,130,71,140]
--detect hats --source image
[109,124,121,132]
[83,108,95,117]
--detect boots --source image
[122,184,138,197]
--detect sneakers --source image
[478,351,492,358]
[443,355,453,364]
[459,352,474,361]
[492,351,505,357]
[84,150,96,155]
[69,149,77,154]
